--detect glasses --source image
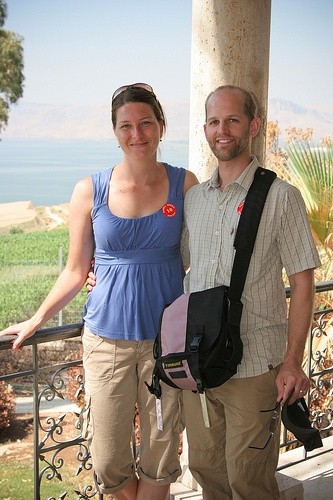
[247,399,282,454]
[109,83,155,99]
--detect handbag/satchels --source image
[145,284,246,398]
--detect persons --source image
[85,85,322,500]
[0,83,200,500]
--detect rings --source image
[300,389,307,394]
